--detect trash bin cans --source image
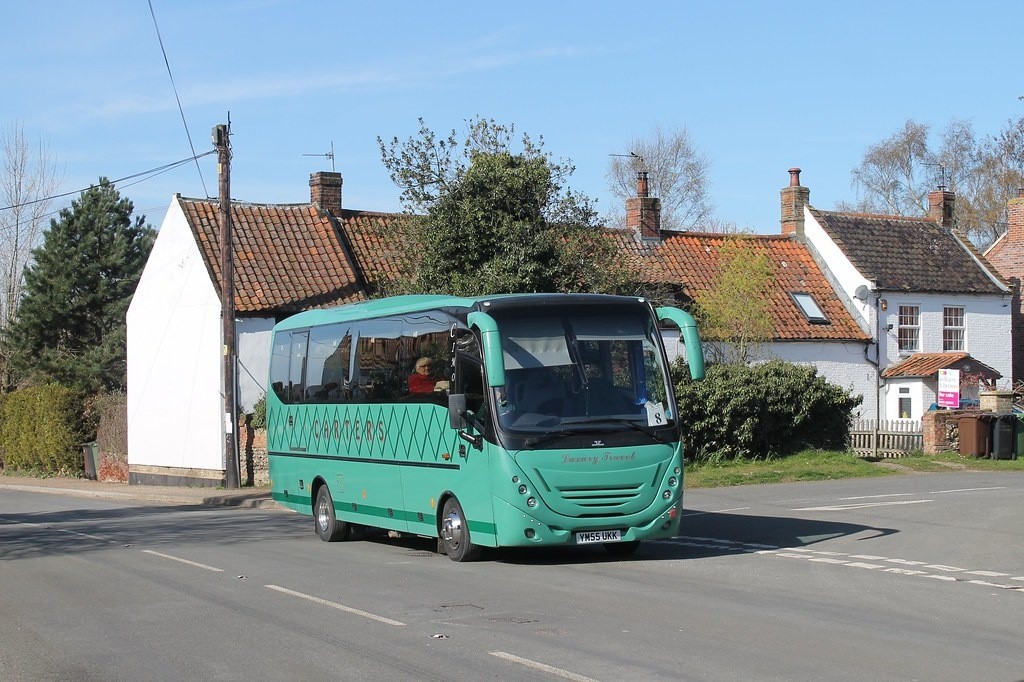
[955,413,991,460]
[80,440,96,481]
[991,414,1024,460]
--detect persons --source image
[407,357,443,394]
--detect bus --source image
[266,293,706,563]
